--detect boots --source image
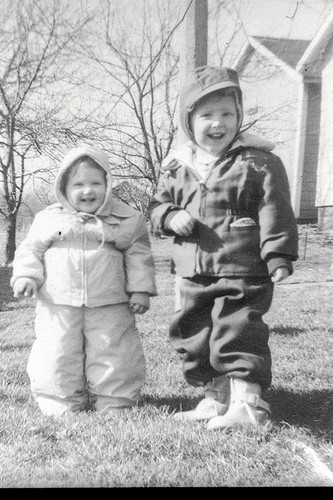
[209,378,271,430]
[171,376,226,422]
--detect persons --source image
[151,65,299,430]
[10,145,158,416]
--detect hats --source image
[53,143,113,219]
[178,64,243,153]
